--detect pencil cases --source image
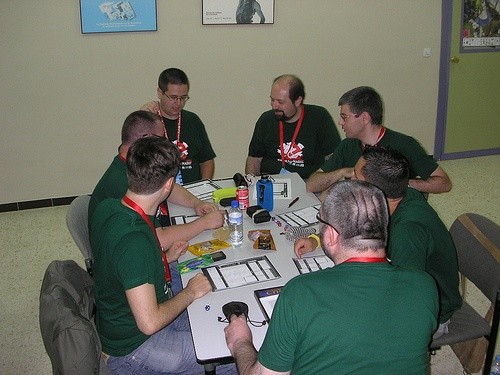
[246,205,271,224]
[233,173,248,187]
[213,187,238,203]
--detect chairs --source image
[429,213,500,375]
[66,196,95,275]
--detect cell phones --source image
[208,251,226,262]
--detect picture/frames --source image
[79,0,158,34]
[201,0,275,25]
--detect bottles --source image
[227,200,243,248]
[256,175,273,212]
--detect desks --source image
[39,260,115,375]
[167,172,335,375]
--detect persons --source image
[305,86,452,203]
[139,67,217,184]
[223,181,440,375]
[89,133,241,375]
[244,74,341,181]
[88,110,227,252]
[293,144,462,339]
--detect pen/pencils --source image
[286,197,299,208]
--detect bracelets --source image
[307,233,321,252]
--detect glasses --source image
[315,212,340,235]
[340,113,362,122]
[161,89,189,102]
